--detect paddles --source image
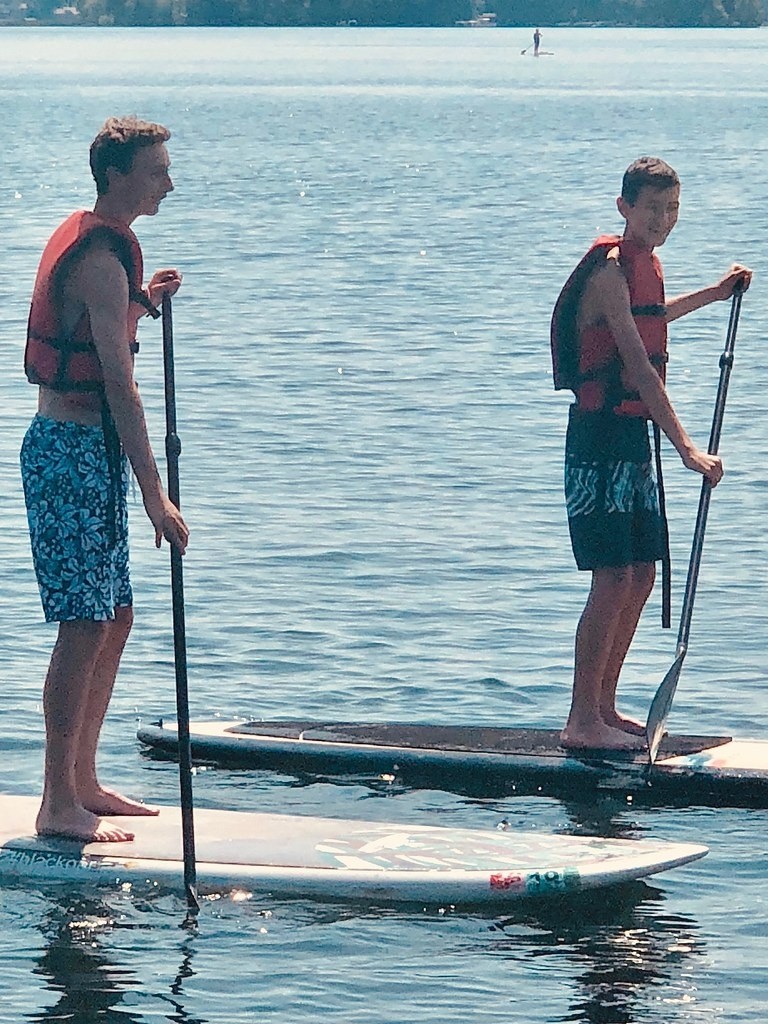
[644,267,745,766]
[161,273,201,910]
[520,36,542,54]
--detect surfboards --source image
[134,717,768,781]
[0,793,711,905]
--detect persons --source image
[19,116,189,840]
[549,158,752,750]
[533,28,542,54]
